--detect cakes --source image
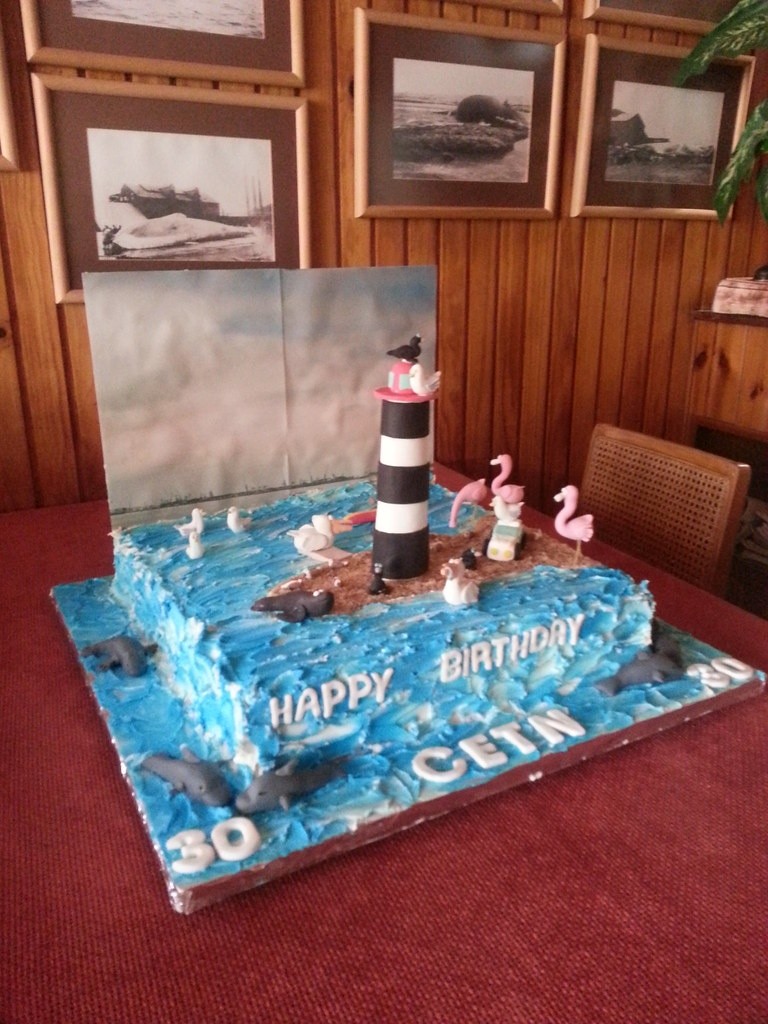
[51,335,753,890]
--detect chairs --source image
[578,423,750,598]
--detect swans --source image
[448,453,594,570]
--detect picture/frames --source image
[31,71,313,304]
[19,0,307,90]
[354,6,568,224]
[570,33,756,220]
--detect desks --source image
[0,460,768,1024]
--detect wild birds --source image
[175,506,335,561]
[438,560,479,604]
